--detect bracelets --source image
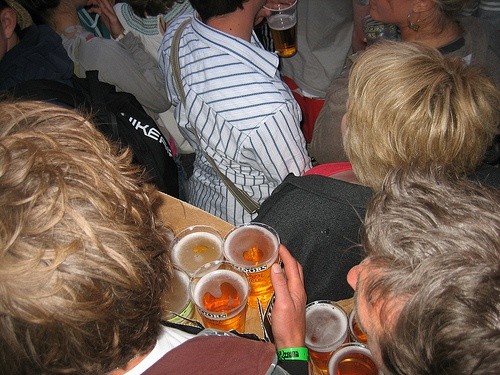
[277,347,308,362]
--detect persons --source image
[0,97,310,375]
[0,0,500,344]
[345,155,500,375]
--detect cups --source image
[349,308,368,347]
[327,343,387,375]
[304,300,348,375]
[168,224,226,278]
[263,0,298,58]
[160,265,195,327]
[223,224,280,309]
[361,15,398,45]
[188,261,249,334]
